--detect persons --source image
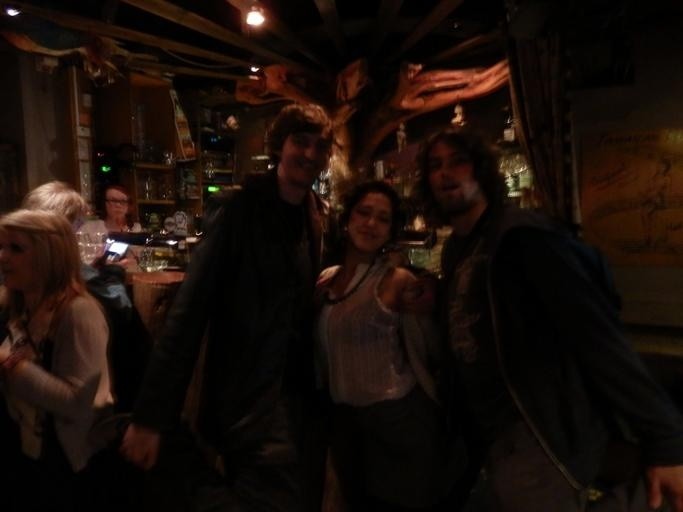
[414,129,682,512]
[122,101,438,512]
[314,179,446,511]
[0,178,157,510]
[381,238,440,282]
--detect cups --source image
[140,246,169,273]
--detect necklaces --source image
[322,263,373,305]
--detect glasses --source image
[106,199,128,205]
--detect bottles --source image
[503,107,516,142]
[170,89,197,162]
